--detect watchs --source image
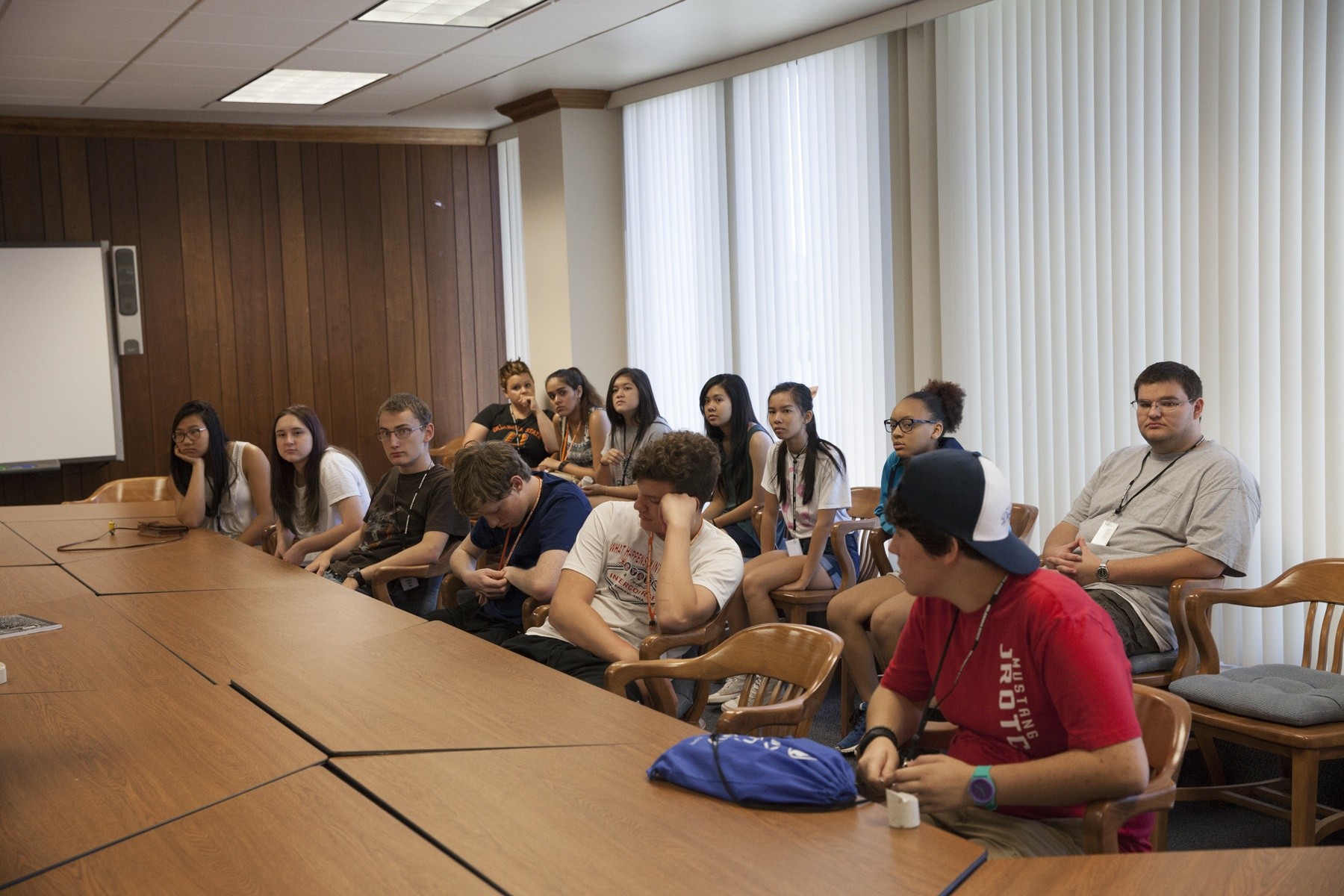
[1095,559,1111,582]
[348,568,366,587]
[968,764,999,812]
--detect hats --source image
[898,451,1040,576]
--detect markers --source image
[12,464,36,468]
[0,466,8,470]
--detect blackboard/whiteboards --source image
[0,240,124,473]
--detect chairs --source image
[263,436,1343,853]
[63,477,171,504]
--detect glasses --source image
[884,418,939,433]
[376,427,427,441]
[1130,397,1198,413]
[172,428,206,443]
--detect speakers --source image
[111,244,145,355]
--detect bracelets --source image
[606,485,608,496]
[558,460,568,472]
[856,726,900,757]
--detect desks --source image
[949,845,1344,896]
[0,499,988,896]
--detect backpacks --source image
[647,733,858,811]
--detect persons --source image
[834,450,1153,855]
[166,358,965,756]
[1039,362,1264,662]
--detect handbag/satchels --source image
[137,521,189,538]
